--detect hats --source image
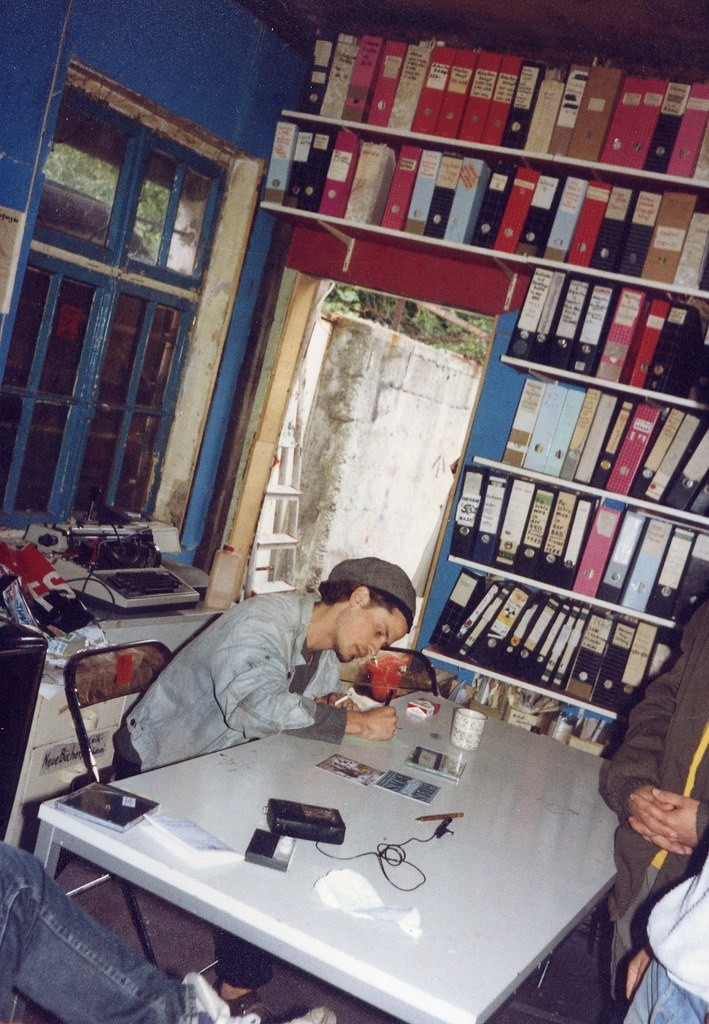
[327,557,417,617]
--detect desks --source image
[32,690,616,1024]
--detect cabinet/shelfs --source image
[259,104,709,756]
[1,600,227,851]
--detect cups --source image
[450,708,487,752]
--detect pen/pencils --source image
[385,689,395,707]
[415,812,464,820]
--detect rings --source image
[394,729,398,734]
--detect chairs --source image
[52,641,219,978]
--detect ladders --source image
[243,360,307,600]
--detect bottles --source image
[204,545,241,609]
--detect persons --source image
[0,839,337,1024]
[110,555,420,1024]
[600,595,709,1024]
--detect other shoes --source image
[211,977,271,1024]
[283,1008,338,1024]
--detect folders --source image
[261,22,708,707]
